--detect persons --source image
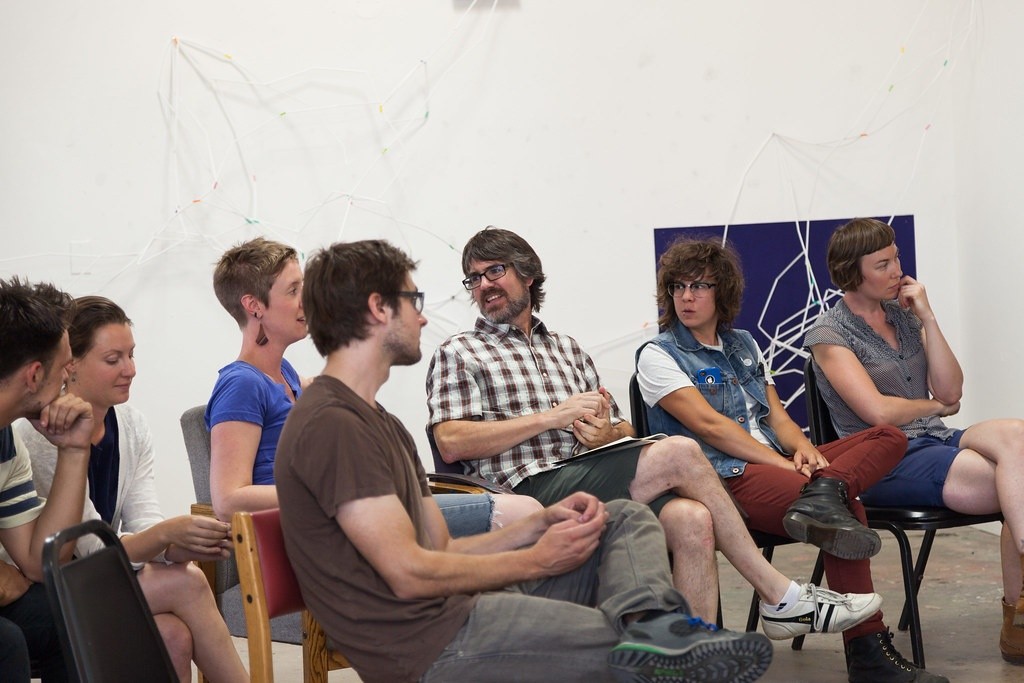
[0,280,96,683]
[271,239,773,683]
[12,295,251,683]
[635,237,950,683]
[802,219,1024,662]
[204,239,310,523]
[426,228,885,641]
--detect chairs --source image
[629,357,1004,671]
[42,518,180,683]
[180,406,357,683]
[418,427,515,497]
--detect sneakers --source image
[607,608,773,683]
[758,580,883,641]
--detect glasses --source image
[668,277,717,298]
[463,262,514,290]
[376,291,425,314]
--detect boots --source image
[1000,550,1024,666]
[783,475,881,560]
[847,627,948,682]
